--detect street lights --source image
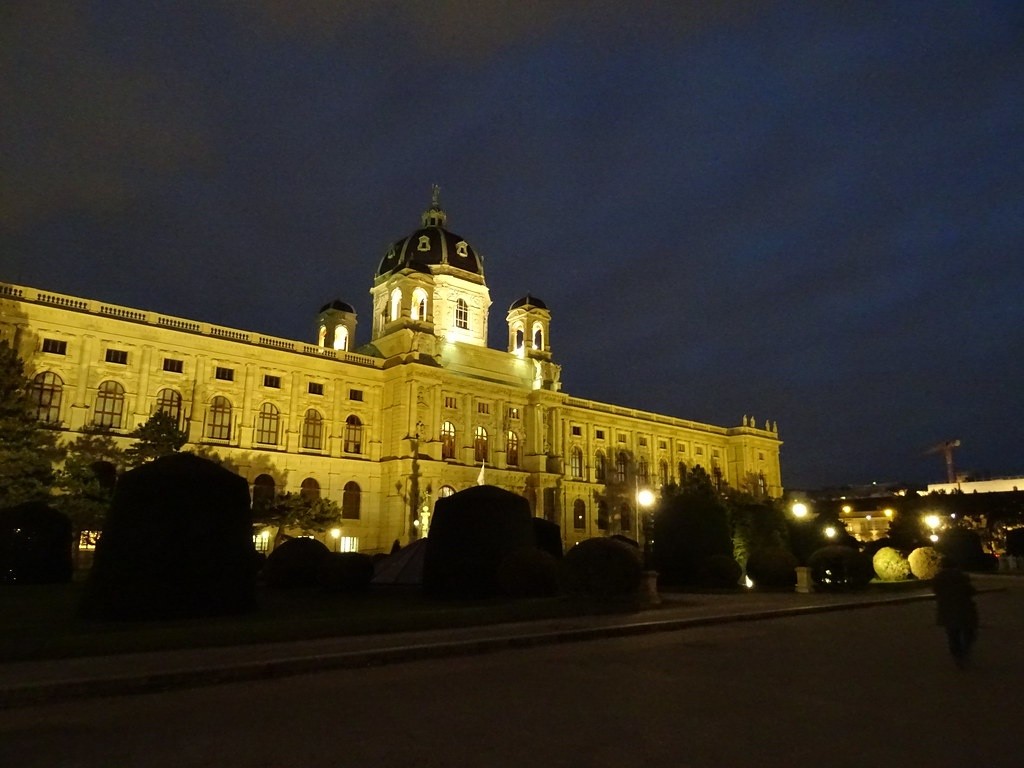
[634,475,652,543]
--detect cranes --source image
[925,438,961,483]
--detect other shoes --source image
[956,662,976,673]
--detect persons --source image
[931,559,980,676]
